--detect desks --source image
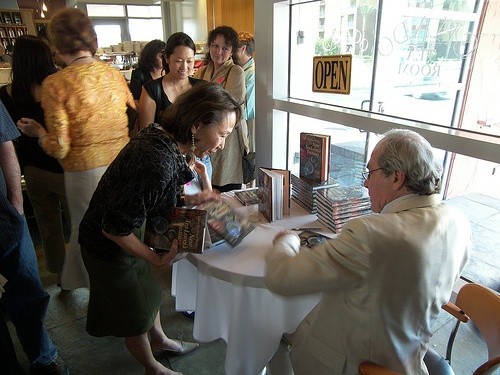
[171,184,340,375]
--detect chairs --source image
[359,282,500,375]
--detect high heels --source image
[149,339,199,358]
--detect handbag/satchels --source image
[242,152,255,184]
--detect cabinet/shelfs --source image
[0,8,39,55]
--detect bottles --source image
[0,13,24,50]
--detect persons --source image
[181,152,214,192]
[233,32,256,191]
[126,39,168,111]
[263,128,467,375]
[192,26,247,196]
[0,97,67,375]
[0,39,14,68]
[16,8,138,298]
[0,35,72,296]
[77,82,243,375]
[134,31,214,190]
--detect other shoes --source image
[40,356,68,375]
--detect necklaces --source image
[70,56,94,65]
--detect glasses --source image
[361,166,385,181]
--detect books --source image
[94,54,140,73]
[0,27,28,39]
[191,59,206,75]
[0,10,25,25]
[95,40,152,54]
[1,39,15,55]
[144,131,380,255]
[195,42,210,54]
